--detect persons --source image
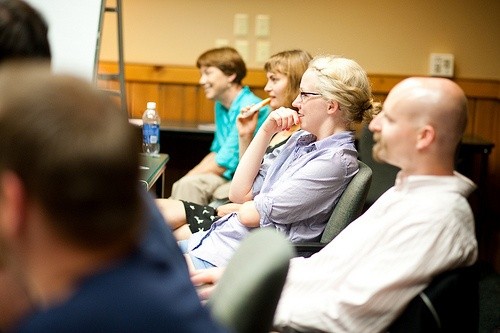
[1,0,52,67]
[190,76,479,332]
[174,52,382,287]
[151,48,314,241]
[0,61,221,332]
[169,45,274,207]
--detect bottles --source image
[142,102,160,154]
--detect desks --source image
[138,153,169,198]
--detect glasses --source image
[297,88,322,102]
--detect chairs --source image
[290,158,373,252]
[208,226,293,333]
